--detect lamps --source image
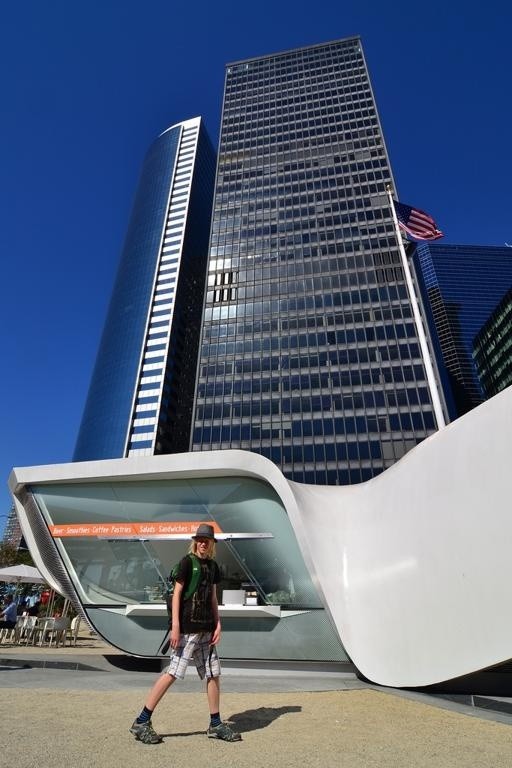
[0,611,81,650]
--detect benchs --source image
[390,199,444,241]
[0,563,48,584]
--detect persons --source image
[0,581,65,644]
[128,523,241,742]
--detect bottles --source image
[192,524,219,543]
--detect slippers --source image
[165,554,216,625]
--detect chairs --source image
[129,717,161,743]
[208,722,241,742]
